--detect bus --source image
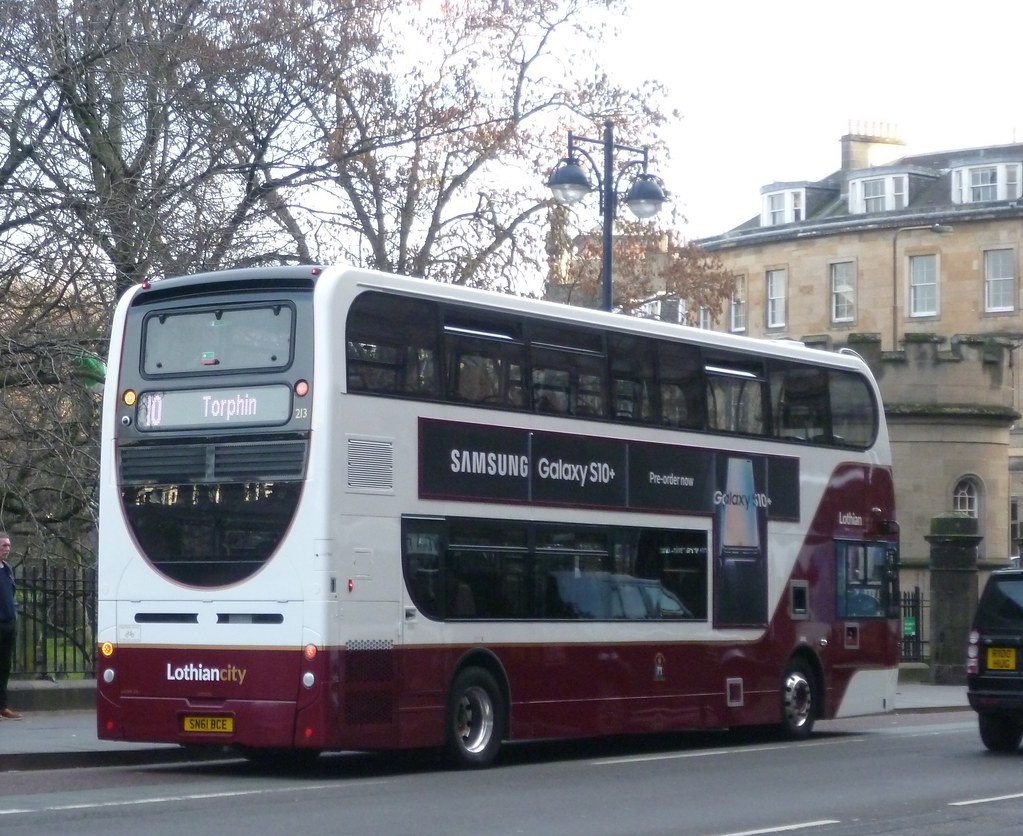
[95,261,906,769]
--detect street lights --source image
[547,119,666,315]
[891,223,956,355]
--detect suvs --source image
[965,566,1023,753]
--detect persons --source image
[0,532,23,721]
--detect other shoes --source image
[0,708,22,720]
